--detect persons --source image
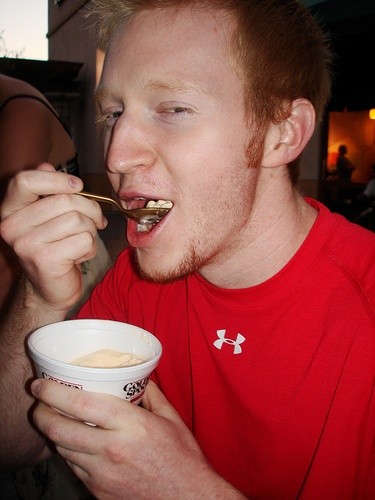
[333,144,356,209]
[0,0,375,500]
[0,70,114,315]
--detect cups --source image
[27,317,163,429]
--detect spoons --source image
[43,188,171,225]
